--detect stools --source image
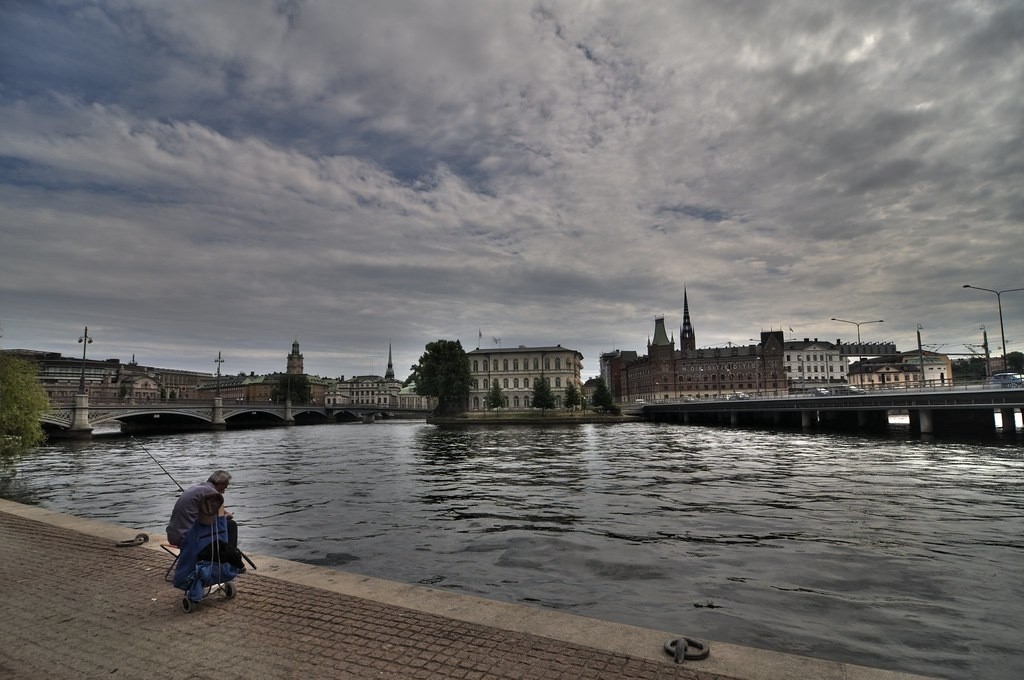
[160,543,181,583]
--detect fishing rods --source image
[129,436,258,570]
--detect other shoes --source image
[236,567,246,574]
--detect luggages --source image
[172,514,236,613]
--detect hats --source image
[196,493,224,525]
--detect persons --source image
[166,470,246,574]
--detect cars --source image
[683,396,694,402]
[984,373,1024,388]
[635,399,646,406]
[814,388,830,396]
[849,386,867,395]
[732,392,750,400]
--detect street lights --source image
[128,354,138,406]
[798,356,805,397]
[916,323,926,388]
[655,382,661,404]
[583,396,586,416]
[482,399,485,418]
[700,368,703,403]
[979,324,990,376]
[214,351,224,398]
[963,284,1024,373]
[75,326,93,395]
[831,318,884,394]
[727,369,731,402]
[755,356,760,400]
